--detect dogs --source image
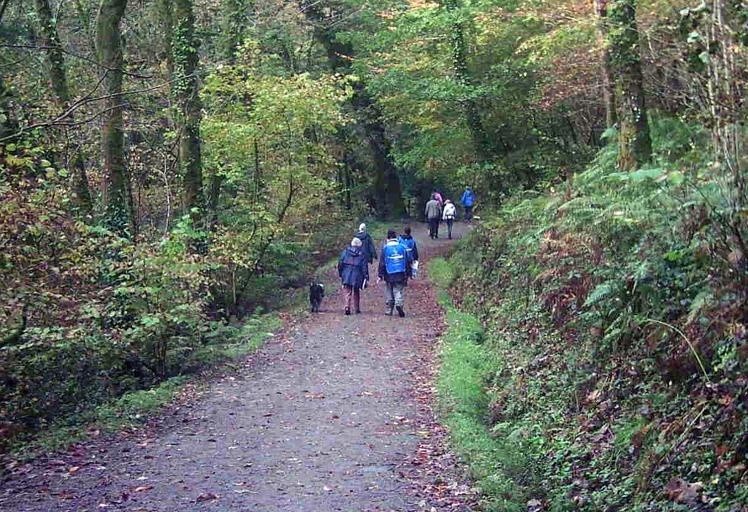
[308,281,325,313]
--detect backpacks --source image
[384,236,415,274]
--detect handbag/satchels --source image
[410,260,419,279]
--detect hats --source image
[359,223,366,232]
[444,199,450,204]
[351,237,363,247]
[388,230,396,239]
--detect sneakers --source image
[345,306,360,315]
[384,305,405,317]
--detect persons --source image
[337,222,420,318]
[459,185,478,224]
[425,186,457,239]
